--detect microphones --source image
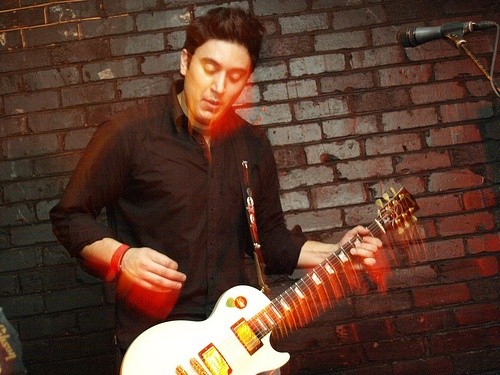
[396,19,495,47]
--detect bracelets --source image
[118,246,133,272]
[106,244,130,282]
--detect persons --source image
[46,2,382,375]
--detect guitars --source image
[120,186,421,375]
